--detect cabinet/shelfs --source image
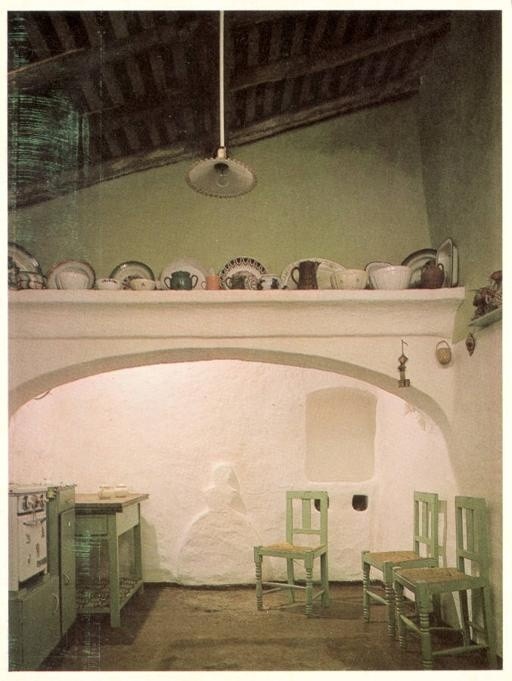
[73,493,149,629]
[9,483,78,670]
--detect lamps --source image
[186,9,257,199]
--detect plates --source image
[8,238,461,290]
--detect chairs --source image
[393,496,497,669]
[253,489,331,614]
[362,489,445,640]
